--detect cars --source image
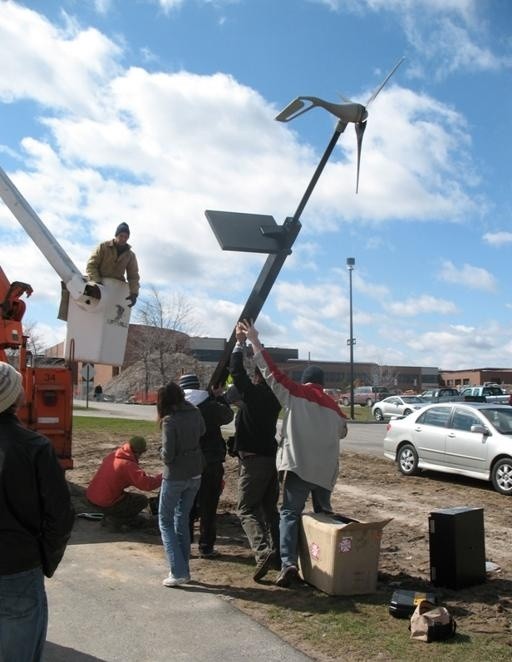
[382,398,512,495]
[372,394,425,422]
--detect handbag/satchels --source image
[409,600,456,643]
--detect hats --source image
[115,223,130,237]
[302,367,323,384]
[130,436,146,453]
[0,360,23,412]
[180,373,200,390]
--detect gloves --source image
[126,294,136,307]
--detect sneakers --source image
[163,577,190,588]
[275,565,297,586]
[253,551,274,580]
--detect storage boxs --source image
[297,509,395,596]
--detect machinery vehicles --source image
[0,169,132,478]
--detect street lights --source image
[344,254,358,420]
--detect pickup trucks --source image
[342,382,391,406]
[420,389,487,403]
[462,386,512,404]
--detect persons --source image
[83,435,162,532]
[84,219,139,307]
[227,324,287,580]
[0,361,76,661]
[238,316,349,587]
[177,372,233,557]
[154,380,206,588]
